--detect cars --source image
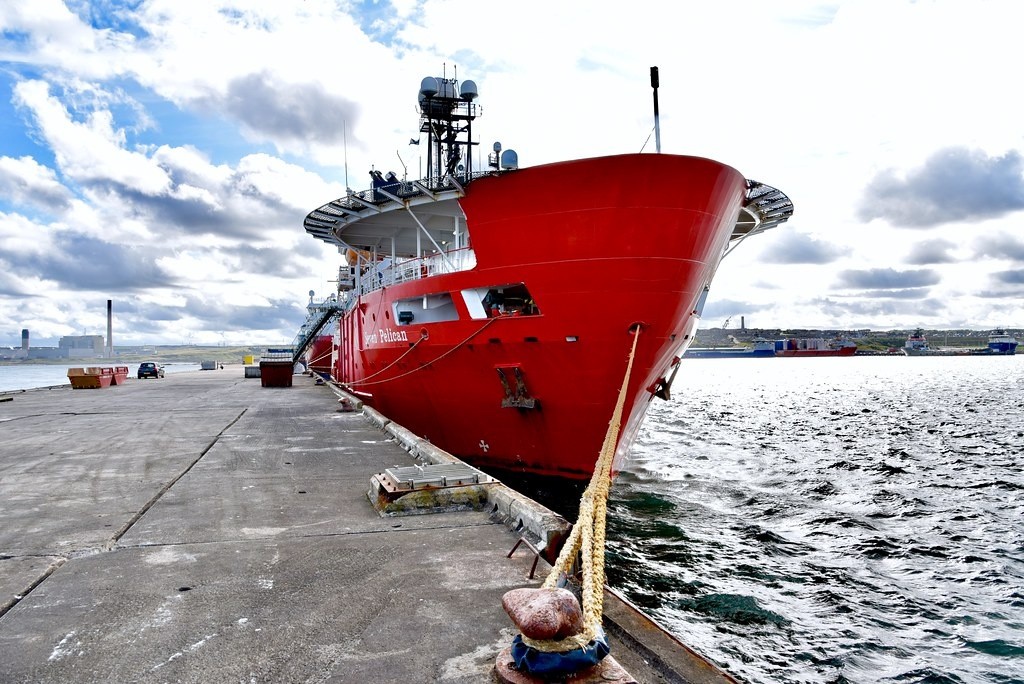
[137,362,165,379]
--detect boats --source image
[987,336,1018,354]
[900,345,943,355]
[777,344,858,357]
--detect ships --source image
[298,289,338,380]
[296,59,797,520]
